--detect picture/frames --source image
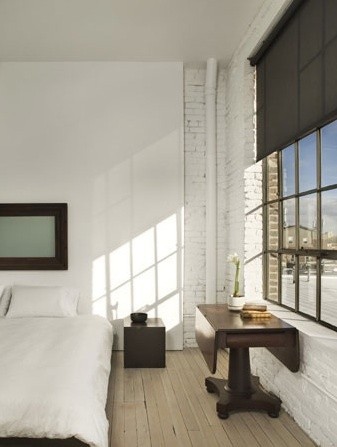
[0,203,68,270]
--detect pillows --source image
[0,288,11,317]
[4,287,83,318]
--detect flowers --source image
[228,252,240,297]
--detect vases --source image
[227,297,246,307]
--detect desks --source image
[195,303,300,419]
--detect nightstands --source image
[124,318,166,368]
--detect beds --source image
[0,315,119,447]
